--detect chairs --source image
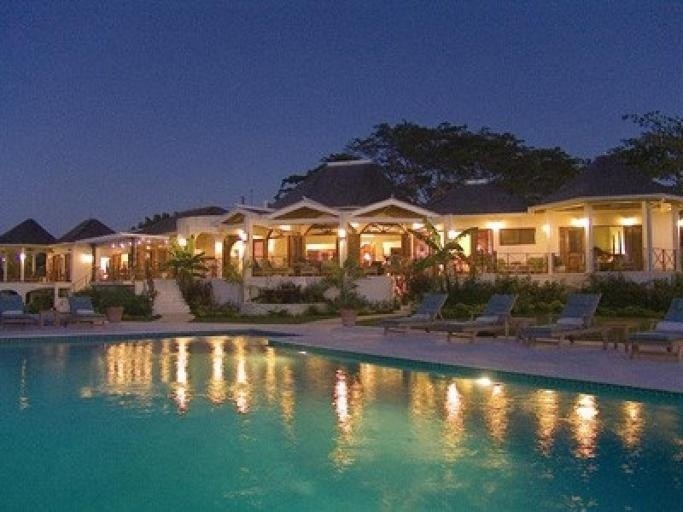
[528,293,603,349]
[382,293,448,336]
[447,294,519,342]
[1,291,108,330]
[628,298,683,360]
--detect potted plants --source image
[102,285,135,323]
[334,288,370,327]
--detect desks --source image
[603,320,640,350]
[509,317,537,342]
